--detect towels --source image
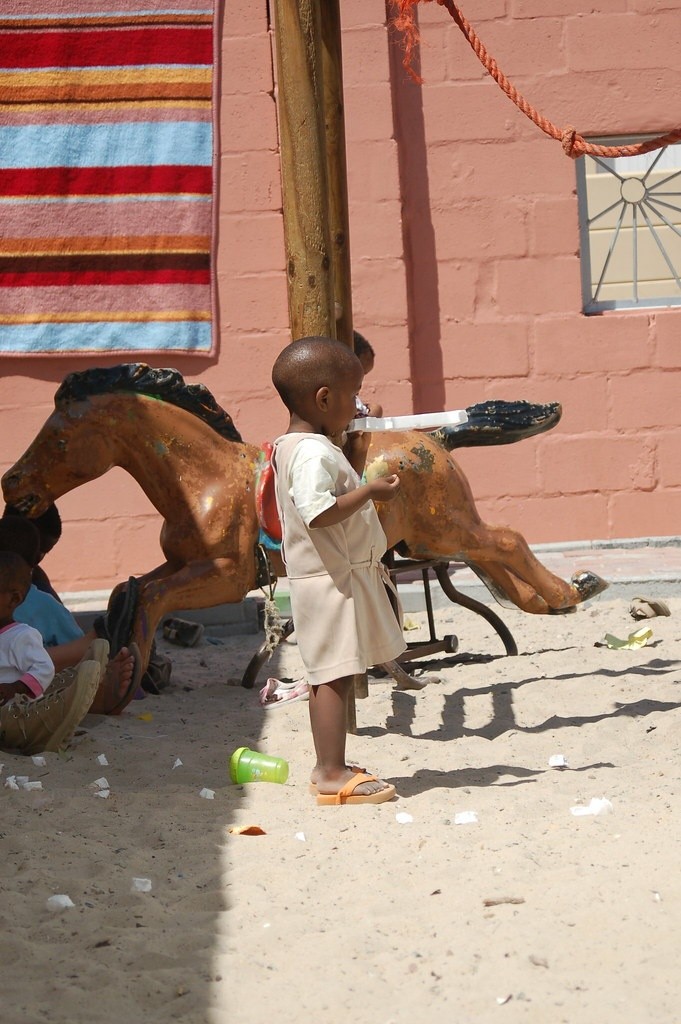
[0,0,229,386]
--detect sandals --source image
[630,594,671,621]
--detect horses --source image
[1,362,609,700]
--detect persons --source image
[0,497,144,755]
[271,336,400,804]
[352,330,383,418]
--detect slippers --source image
[308,761,378,796]
[316,773,396,806]
[93,574,139,656]
[103,642,144,715]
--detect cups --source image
[229,746,289,783]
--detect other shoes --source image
[257,673,309,710]
[161,617,204,648]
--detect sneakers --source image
[44,637,110,697]
[0,659,99,756]
